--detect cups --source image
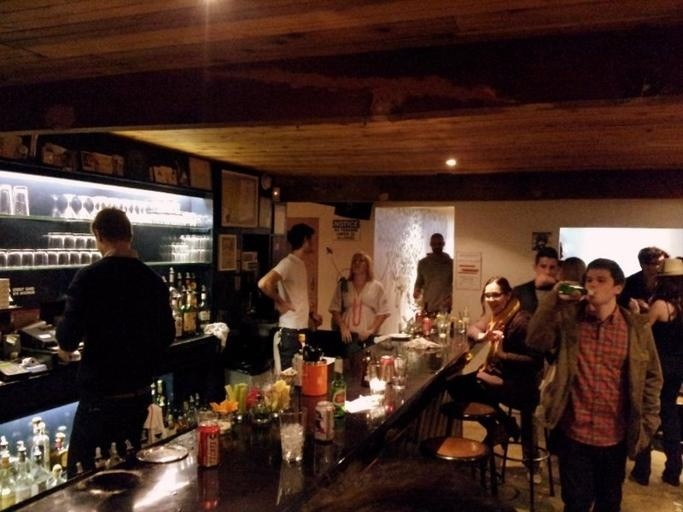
[291,352,328,396]
[14,186,29,215]
[0,183,11,214]
[275,406,308,465]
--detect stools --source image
[438,398,500,493]
[499,387,557,510]
[418,434,505,511]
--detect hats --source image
[651,257,683,281]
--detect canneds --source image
[197,422,220,468]
[379,356,394,384]
[423,317,432,336]
[314,401,336,442]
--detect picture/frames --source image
[218,229,238,273]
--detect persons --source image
[617,247,683,488]
[412,232,453,318]
[255,221,324,377]
[326,251,392,355]
[447,276,531,447]
[512,246,586,315]
[53,207,176,487]
[523,256,665,512]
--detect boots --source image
[472,407,521,451]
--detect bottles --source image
[360,347,408,389]
[558,283,596,297]
[0,378,200,511]
[0,230,101,269]
[405,308,469,337]
[330,357,347,419]
[163,267,215,338]
[228,394,273,463]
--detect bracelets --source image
[307,309,315,317]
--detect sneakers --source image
[526,469,543,484]
[627,471,650,487]
[661,472,681,486]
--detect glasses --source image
[484,292,504,297]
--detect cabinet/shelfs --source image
[0,160,214,292]
[0,324,220,487]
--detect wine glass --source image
[171,233,209,261]
[48,192,209,226]
